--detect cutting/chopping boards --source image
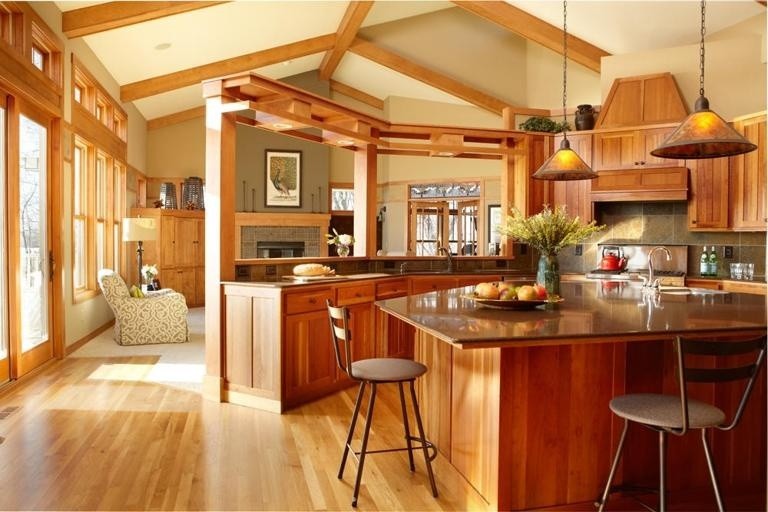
[280,275,346,282]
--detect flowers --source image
[496,202,608,246]
[140,263,160,281]
[324,226,358,248]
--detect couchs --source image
[95,266,192,345]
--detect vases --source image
[532,244,562,312]
[337,243,351,258]
[572,101,598,133]
[147,279,155,291]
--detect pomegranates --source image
[473,283,547,300]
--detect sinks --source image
[657,286,729,296]
[407,270,445,275]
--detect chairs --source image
[591,329,767,511]
[323,295,442,511]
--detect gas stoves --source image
[587,269,684,282]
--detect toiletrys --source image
[729,263,754,280]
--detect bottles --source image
[575,104,594,131]
[699,244,708,276]
[709,244,718,276]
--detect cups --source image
[741,264,754,281]
[729,263,742,279]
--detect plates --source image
[460,292,563,309]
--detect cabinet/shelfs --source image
[377,275,414,362]
[335,278,376,395]
[130,207,204,310]
[553,111,767,234]
[220,280,336,414]
[233,211,332,258]
[409,274,501,295]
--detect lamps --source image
[122,215,161,285]
[528,0,604,182]
[650,0,762,162]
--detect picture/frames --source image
[263,146,305,209]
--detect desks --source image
[373,277,767,510]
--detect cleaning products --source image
[699,245,720,278]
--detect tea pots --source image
[601,246,627,272]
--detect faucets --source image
[638,288,670,332]
[437,245,454,272]
[639,246,672,288]
